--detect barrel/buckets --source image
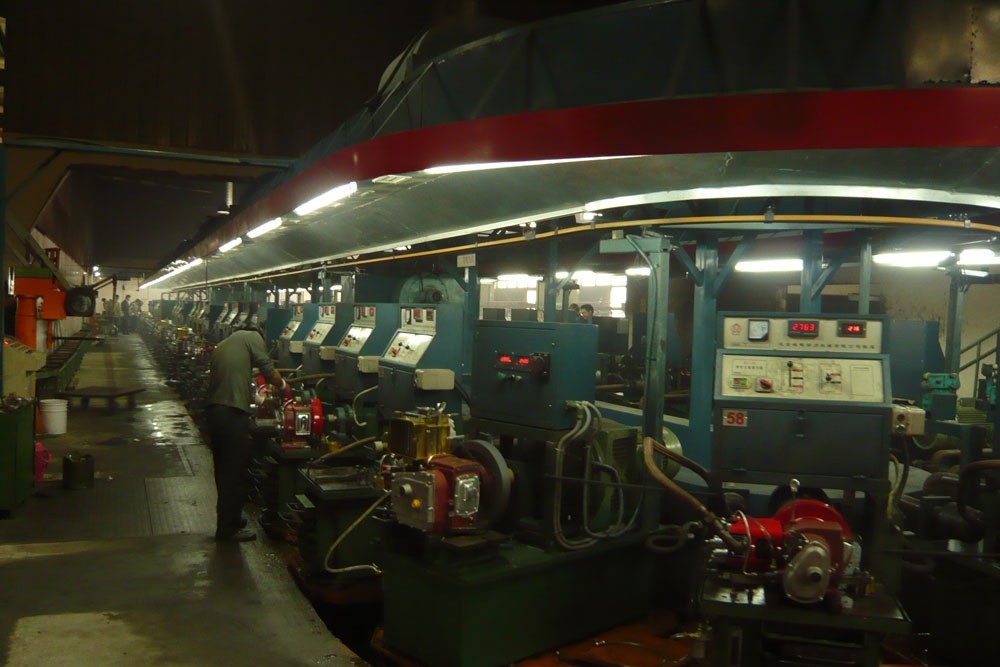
[35,398,68,435]
[63,454,95,489]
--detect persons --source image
[206,327,286,543]
[575,304,600,324]
[102,295,120,321]
[122,295,130,335]
[567,303,581,319]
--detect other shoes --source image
[233,519,246,528]
[215,529,257,542]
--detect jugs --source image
[35,441,51,483]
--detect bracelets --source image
[279,378,286,390]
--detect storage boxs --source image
[62,454,94,489]
